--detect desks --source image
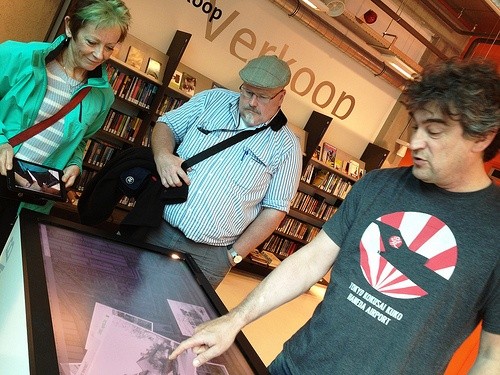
[0,208,269,375]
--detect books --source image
[119,195,137,207]
[300,163,353,200]
[81,137,123,169]
[273,215,321,243]
[262,234,297,258]
[102,108,156,148]
[290,190,339,222]
[72,169,98,193]
[103,62,183,117]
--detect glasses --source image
[239,83,284,103]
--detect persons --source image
[0,0,132,256]
[167,60,500,375]
[136,54,304,294]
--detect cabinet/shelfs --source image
[43,0,390,293]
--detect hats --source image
[239,55,292,89]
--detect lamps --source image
[383,55,419,79]
[299,0,329,12]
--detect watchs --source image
[227,245,243,266]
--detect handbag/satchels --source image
[127,153,189,205]
